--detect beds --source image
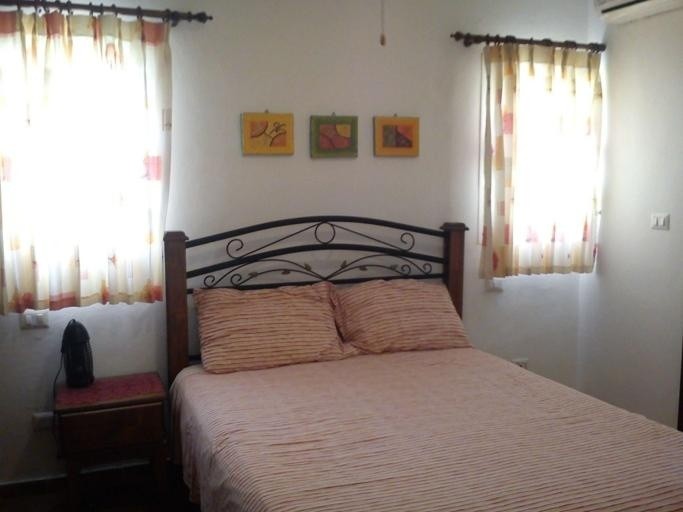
[163,216,683,512]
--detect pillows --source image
[188,281,350,373]
[332,275,472,351]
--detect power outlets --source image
[512,359,528,370]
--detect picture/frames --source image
[374,115,419,156]
[243,112,293,156]
[311,115,359,160]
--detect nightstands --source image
[51,370,171,512]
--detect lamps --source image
[60,320,93,389]
